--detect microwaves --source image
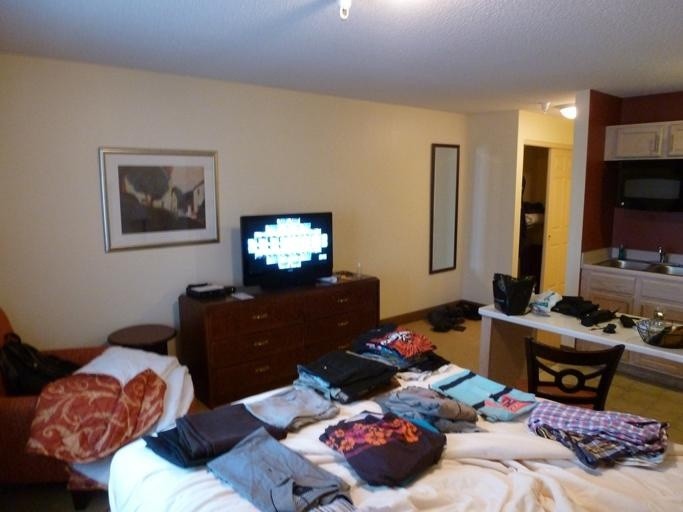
[604,158,683,212]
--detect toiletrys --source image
[617,242,627,259]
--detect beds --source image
[107,363,683,512]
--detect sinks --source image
[593,257,653,271]
[653,263,683,277]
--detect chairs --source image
[524,336,625,411]
[0,307,106,512]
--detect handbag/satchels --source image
[492,271,534,318]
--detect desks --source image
[478,303,683,393]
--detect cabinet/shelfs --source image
[179,272,380,409]
[581,291,634,363]
[614,125,664,159]
[666,122,683,157]
[634,301,683,379]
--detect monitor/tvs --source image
[241,213,333,286]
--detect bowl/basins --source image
[635,320,683,346]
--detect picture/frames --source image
[99,147,220,253]
[429,143,460,274]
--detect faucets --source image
[656,244,669,262]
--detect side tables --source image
[107,324,177,355]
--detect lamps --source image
[560,107,576,120]
